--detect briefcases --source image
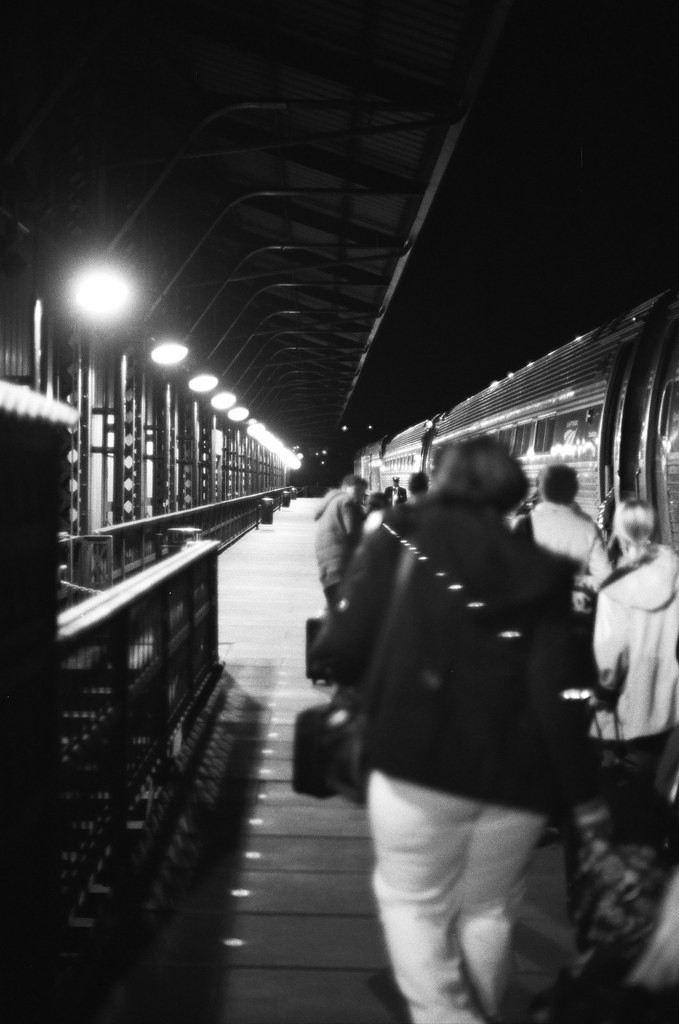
[292,704,342,800]
[305,618,336,685]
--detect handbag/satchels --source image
[320,688,375,805]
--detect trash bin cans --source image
[291,488,297,500]
[167,527,202,554]
[261,498,273,524]
[282,491,290,506]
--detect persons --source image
[306,435,679,1024]
[383,476,407,509]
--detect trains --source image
[352,289,677,558]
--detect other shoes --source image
[534,827,559,848]
